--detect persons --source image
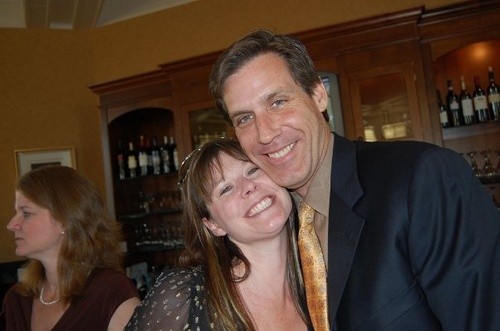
[209,29,500,331]
[0,164,142,331]
[123,137,314,331]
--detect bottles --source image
[446,80,461,128]
[487,67,500,120]
[473,76,489,123]
[364,109,411,141]
[115,135,179,180]
[436,89,449,128]
[459,75,475,125]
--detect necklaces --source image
[39,283,61,306]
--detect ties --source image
[295,200,330,331]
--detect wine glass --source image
[466,150,500,182]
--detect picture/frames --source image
[13,145,78,177]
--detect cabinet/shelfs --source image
[86,0,500,300]
